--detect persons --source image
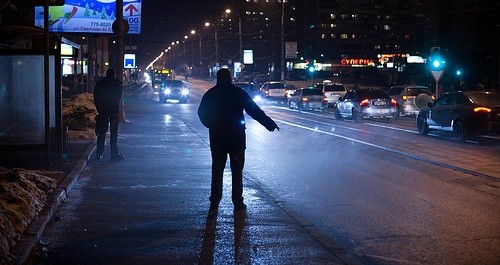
[198,69,279,210]
[438,84,443,95]
[93,68,123,161]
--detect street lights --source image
[205,22,218,72]
[184,35,193,75]
[224,9,244,77]
[191,29,203,79]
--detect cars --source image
[231,74,255,92]
[159,80,189,103]
[332,88,400,122]
[285,84,297,98]
[287,87,330,112]
[416,90,500,138]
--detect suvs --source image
[312,80,347,106]
[385,85,435,117]
[258,80,287,100]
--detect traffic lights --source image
[432,47,441,71]
[455,64,461,76]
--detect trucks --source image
[151,69,175,88]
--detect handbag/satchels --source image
[95,113,108,136]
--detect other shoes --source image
[210,203,218,208]
[234,203,247,210]
[110,153,124,161]
[96,154,104,160]
